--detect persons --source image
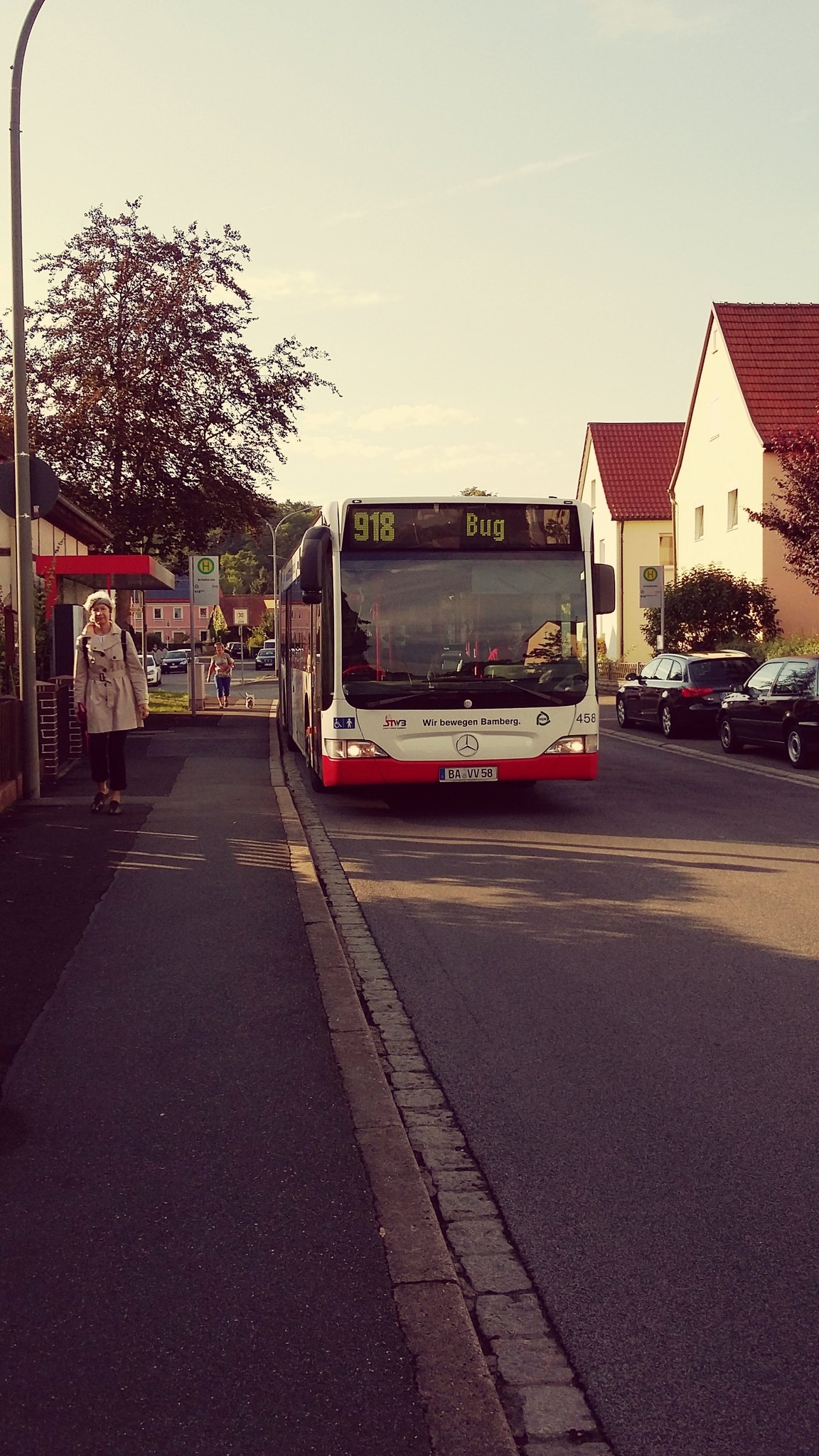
[74,591,149,815]
[488,620,534,666]
[343,566,475,679]
[206,642,235,711]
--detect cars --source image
[224,642,240,651]
[229,643,250,659]
[178,649,200,662]
[255,648,275,671]
[160,650,190,674]
[137,655,161,687]
[717,656,819,769]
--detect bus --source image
[277,496,616,794]
[263,639,275,649]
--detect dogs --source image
[245,692,254,710]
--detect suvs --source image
[616,649,761,739]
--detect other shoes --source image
[224,699,228,707]
[219,704,224,709]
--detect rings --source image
[146,712,149,714]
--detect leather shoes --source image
[109,800,122,815]
[90,788,110,812]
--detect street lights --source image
[255,504,324,676]
